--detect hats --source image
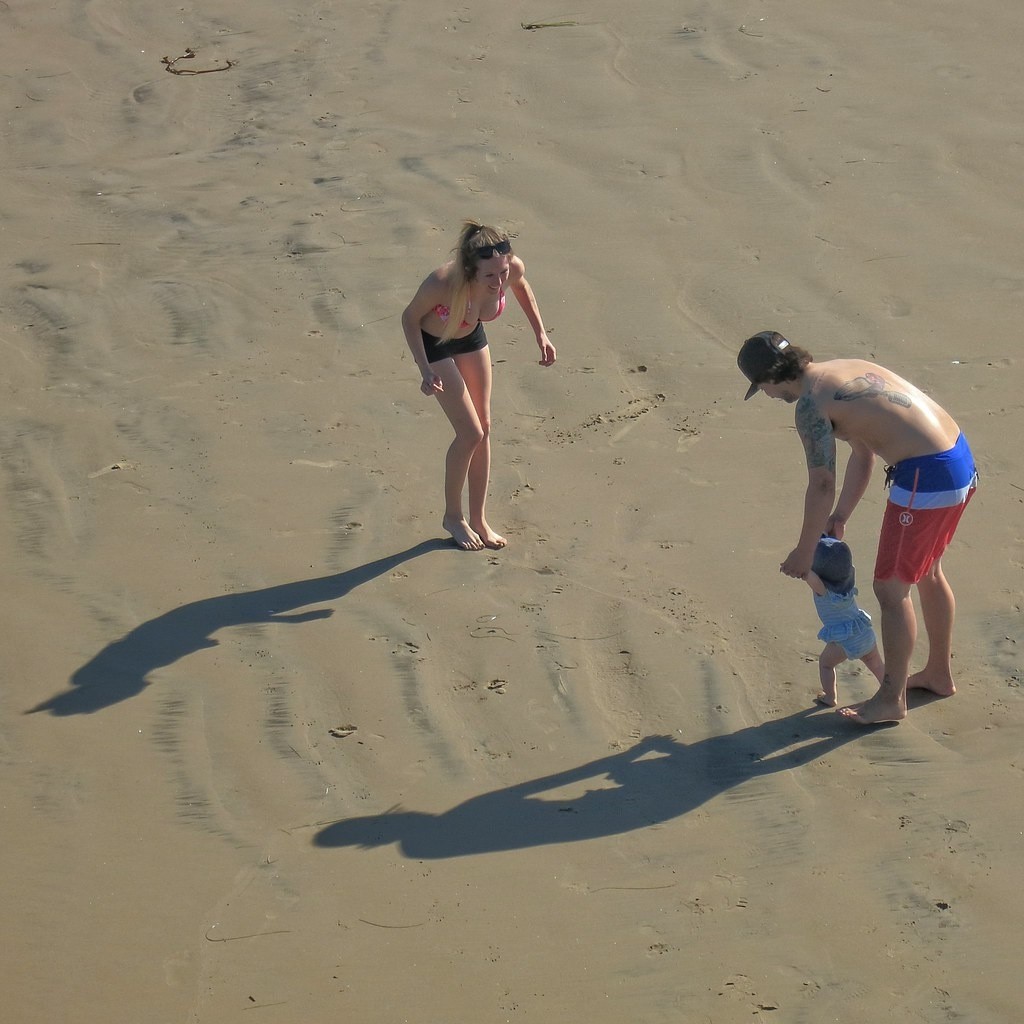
[737,330,791,401]
[810,532,856,595]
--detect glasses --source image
[469,240,511,258]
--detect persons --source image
[734,330,978,726]
[402,220,559,553]
[776,520,885,708]
